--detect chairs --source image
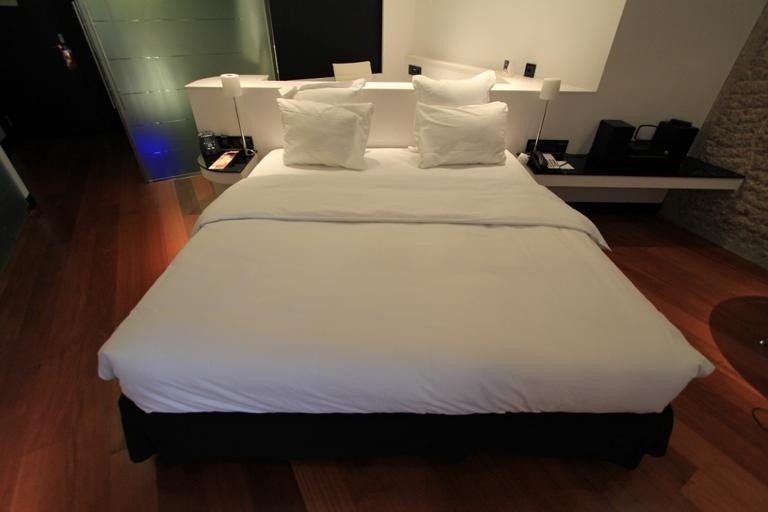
[332,60,373,81]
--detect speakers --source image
[586,120,636,169]
[652,120,700,164]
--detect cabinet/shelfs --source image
[515,139,744,203]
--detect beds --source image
[97,146,716,467]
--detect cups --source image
[198,127,216,150]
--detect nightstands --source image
[197,135,258,197]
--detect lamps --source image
[533,79,561,151]
[221,74,253,156]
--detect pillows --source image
[276,98,374,170]
[409,70,496,153]
[414,101,508,169]
[278,78,367,104]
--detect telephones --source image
[532,151,561,173]
